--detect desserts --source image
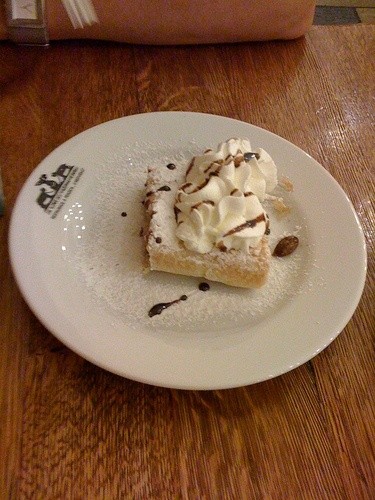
[143,137,279,289]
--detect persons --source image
[0,0,316,45]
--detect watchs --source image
[4,0,50,47]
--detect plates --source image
[8,111,368,392]
